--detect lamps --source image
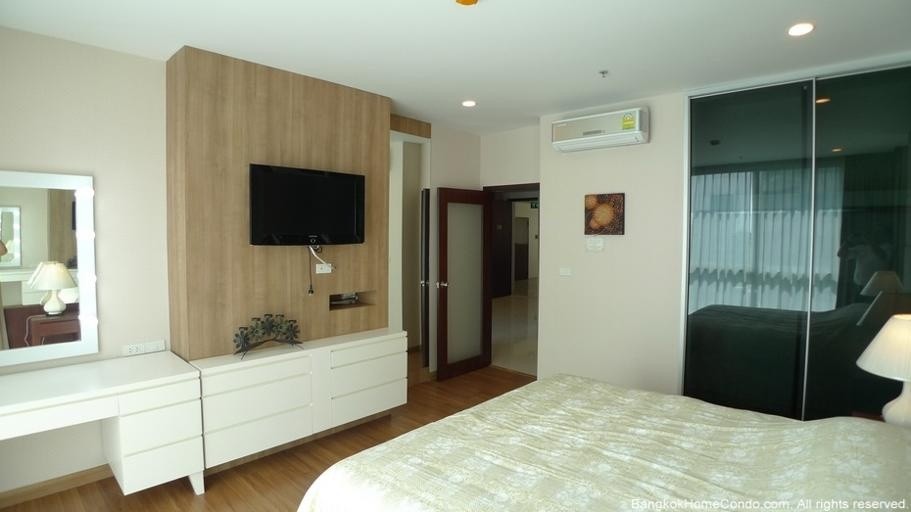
[27,261,77,314]
[858,270,911,331]
[856,315,911,423]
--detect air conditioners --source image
[551,107,649,150]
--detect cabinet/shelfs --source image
[205,354,314,470]
[327,338,407,426]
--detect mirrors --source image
[0,206,23,270]
[0,170,99,367]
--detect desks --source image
[0,351,199,510]
[27,312,81,344]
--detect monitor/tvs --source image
[249,163,365,246]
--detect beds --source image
[684,300,874,413]
[294,371,911,511]
